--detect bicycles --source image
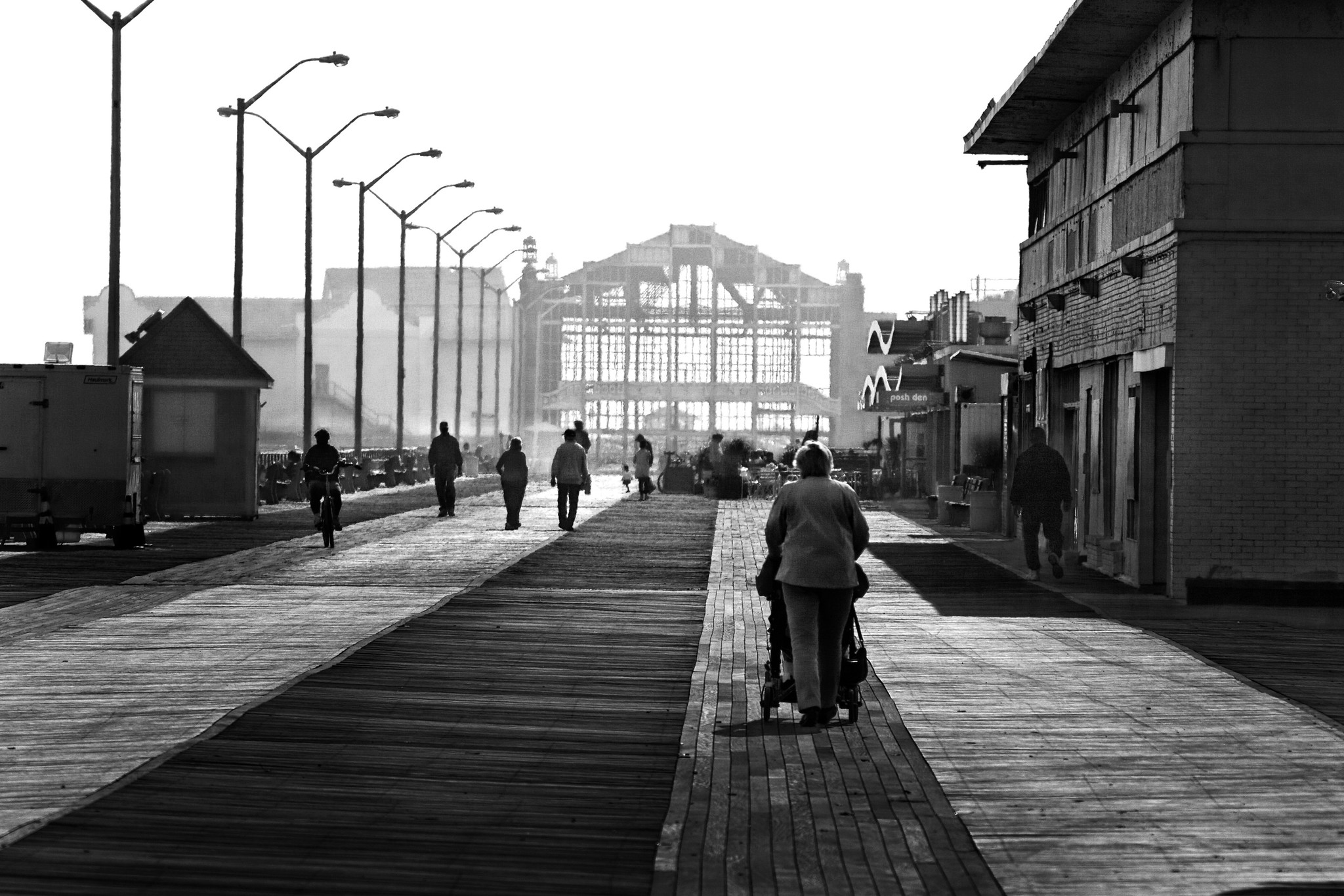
[657,450,685,492]
[300,459,347,548]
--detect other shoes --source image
[639,495,643,500]
[314,517,323,527]
[333,519,342,531]
[437,511,455,517]
[558,523,574,531]
[505,520,521,530]
[644,495,649,500]
[799,702,838,726]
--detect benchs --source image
[926,474,1000,532]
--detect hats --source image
[314,429,330,439]
[562,429,576,439]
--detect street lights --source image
[219,106,400,455]
[232,50,349,346]
[332,147,565,456]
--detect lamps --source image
[1110,100,1138,117]
[1053,147,1078,162]
[1325,280,1344,302]
[1120,255,1143,278]
[1019,306,1035,323]
[1079,277,1099,299]
[1048,294,1065,311]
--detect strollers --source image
[755,547,868,724]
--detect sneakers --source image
[1026,569,1041,581]
[1048,551,1064,578]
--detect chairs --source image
[742,465,883,501]
[257,453,430,506]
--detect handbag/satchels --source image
[644,478,657,494]
[841,648,868,684]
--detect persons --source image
[749,430,869,727]
[428,422,463,517]
[632,434,653,501]
[495,438,529,530]
[621,465,633,493]
[302,429,342,531]
[699,433,724,496]
[461,443,492,474]
[574,420,591,454]
[551,429,588,531]
[1010,427,1073,581]
